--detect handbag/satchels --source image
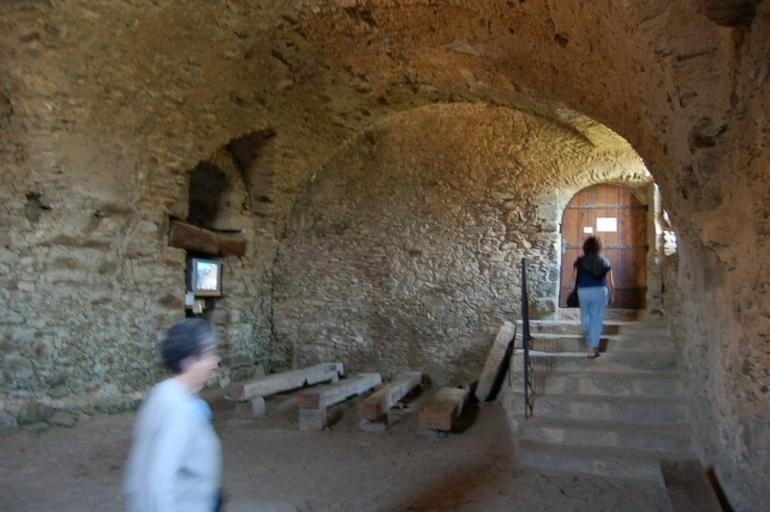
[567,291,579,307]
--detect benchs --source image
[418,386,468,431]
[358,371,422,432]
[295,372,382,432]
[223,362,344,418]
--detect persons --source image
[122,317,224,512]
[571,236,616,359]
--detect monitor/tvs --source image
[187,258,222,298]
